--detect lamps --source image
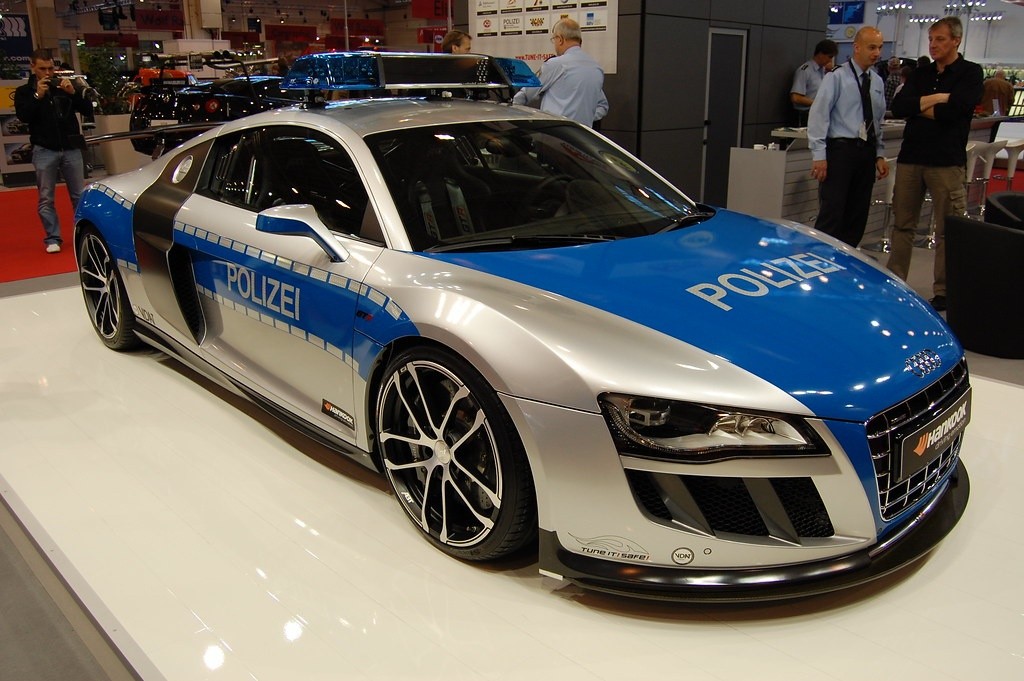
[879,0,1003,23]
[96,6,127,26]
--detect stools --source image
[862,137,1023,251]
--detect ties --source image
[860,72,877,145]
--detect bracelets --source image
[34,92,41,100]
[14,48,94,253]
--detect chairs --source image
[258,140,350,236]
[944,193,1023,361]
[407,140,502,240]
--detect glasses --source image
[550,35,560,43]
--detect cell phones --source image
[48,77,63,86]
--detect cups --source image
[753,144,767,151]
[768,142,779,150]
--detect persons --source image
[591,88,609,134]
[429,29,504,152]
[790,39,1024,145]
[806,26,891,252]
[884,15,985,312]
[511,17,604,180]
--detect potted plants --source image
[76,42,156,174]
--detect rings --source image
[814,169,817,172]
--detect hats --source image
[888,58,900,68]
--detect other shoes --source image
[928,295,948,312]
[46,244,61,253]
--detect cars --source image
[76,53,975,607]
[128,77,307,158]
